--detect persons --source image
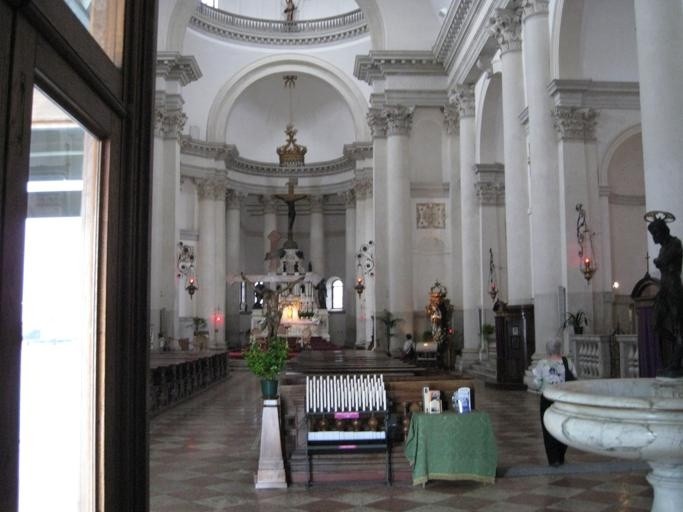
[648,218,683,372]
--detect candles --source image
[302,374,389,412]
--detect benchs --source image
[149,346,240,419]
[288,348,428,384]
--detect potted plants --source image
[565,309,588,333]
[241,334,291,397]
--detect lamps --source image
[575,203,597,286]
[176,241,198,300]
[276,74,305,167]
[352,277,365,299]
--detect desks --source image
[412,409,491,486]
[302,404,393,490]
